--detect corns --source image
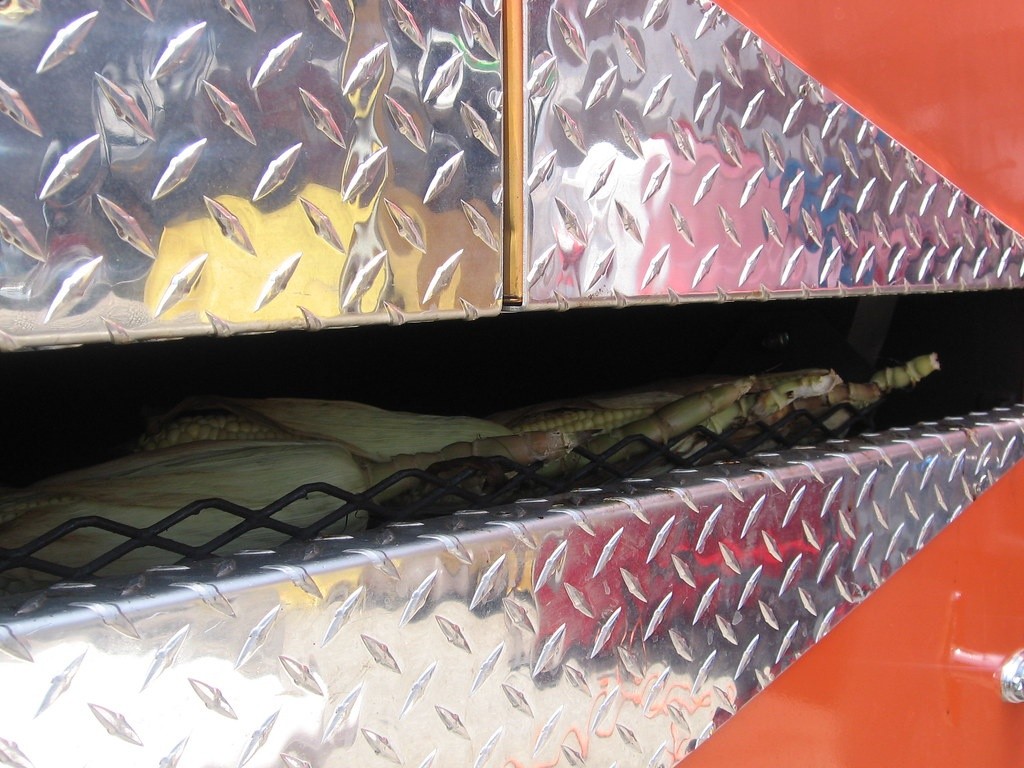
[0,353,946,591]
